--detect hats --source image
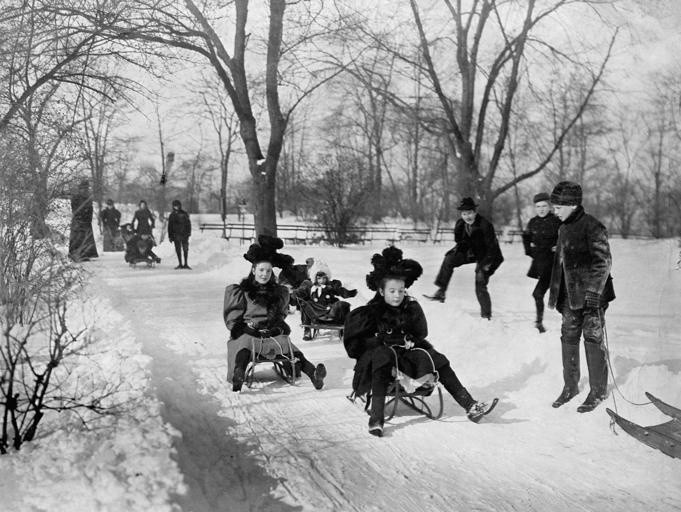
[244,235,294,268]
[534,193,549,202]
[366,246,422,291]
[310,257,330,283]
[458,197,478,210]
[551,182,582,205]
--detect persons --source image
[344,274,498,436]
[423,196,505,318]
[523,193,561,333]
[222,259,327,391]
[70,179,191,270]
[547,182,618,412]
[291,264,358,343]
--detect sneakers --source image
[303,330,311,340]
[233,368,245,391]
[423,290,444,303]
[467,398,498,422]
[369,424,383,437]
[312,364,326,389]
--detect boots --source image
[577,341,607,412]
[552,342,580,408]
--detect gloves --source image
[584,292,601,309]
